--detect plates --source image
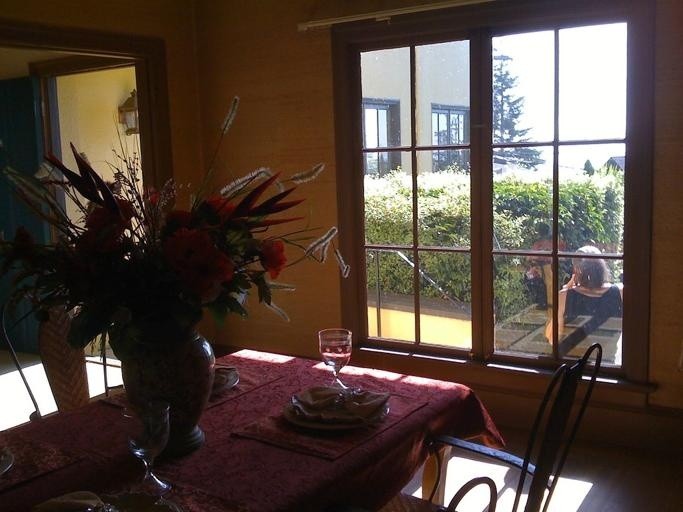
[285,392,392,433]
[33,494,184,512]
[211,364,242,397]
[0,446,16,477]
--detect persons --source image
[548,245,624,346]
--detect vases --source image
[121,333,216,457]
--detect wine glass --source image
[120,399,174,498]
[318,328,362,394]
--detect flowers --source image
[0,95,350,366]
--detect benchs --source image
[494,310,623,364]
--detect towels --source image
[209,363,240,400]
[33,491,104,511]
[291,386,390,424]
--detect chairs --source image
[2,296,123,421]
[378,343,602,512]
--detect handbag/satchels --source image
[435,476,498,510]
[520,263,546,309]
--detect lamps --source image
[118,89,140,136]
